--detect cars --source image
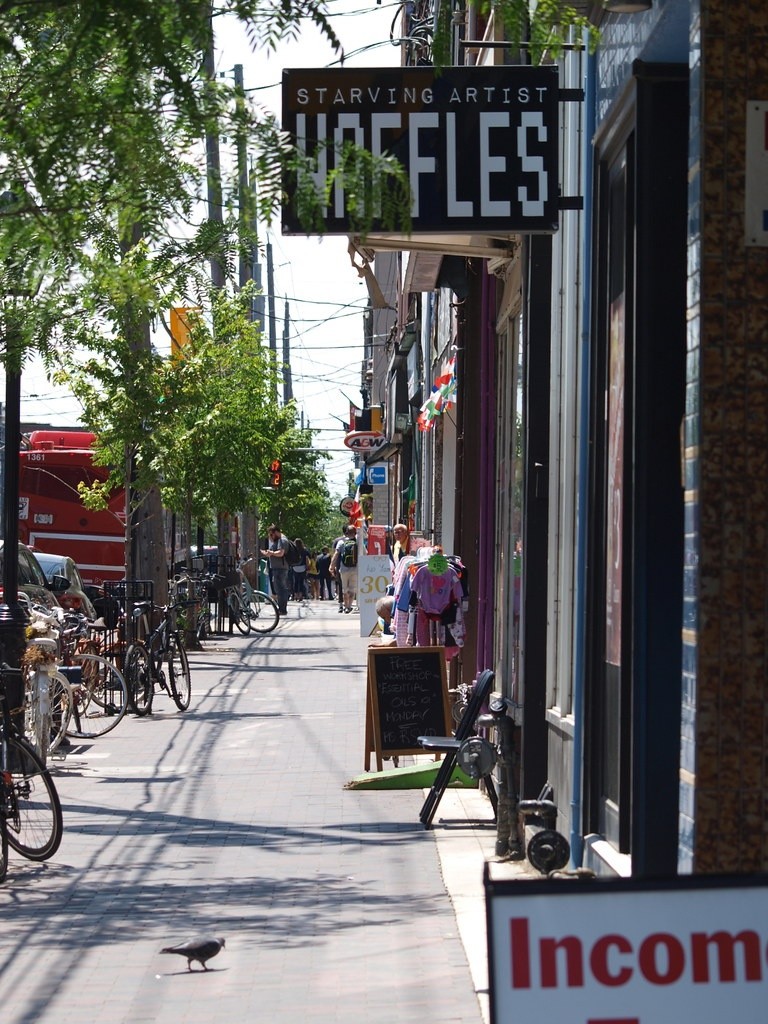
[169,545,222,647]
[0,541,73,693]
[29,552,104,641]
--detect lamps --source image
[602,0,652,13]
[395,413,413,429]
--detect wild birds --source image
[159,938,226,972]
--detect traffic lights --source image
[269,458,282,490]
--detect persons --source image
[261,526,290,616]
[389,524,415,571]
[293,524,358,613]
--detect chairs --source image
[417,669,498,830]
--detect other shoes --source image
[345,607,350,613]
[329,596,334,600]
[277,608,288,615]
[338,607,343,613]
[320,598,324,600]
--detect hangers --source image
[402,546,461,567]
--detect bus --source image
[18,430,126,592]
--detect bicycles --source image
[124,600,201,717]
[0,593,129,884]
[222,558,280,636]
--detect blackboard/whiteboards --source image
[365,646,451,757]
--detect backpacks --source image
[341,537,358,568]
[280,538,300,565]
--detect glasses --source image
[270,532,273,536]
